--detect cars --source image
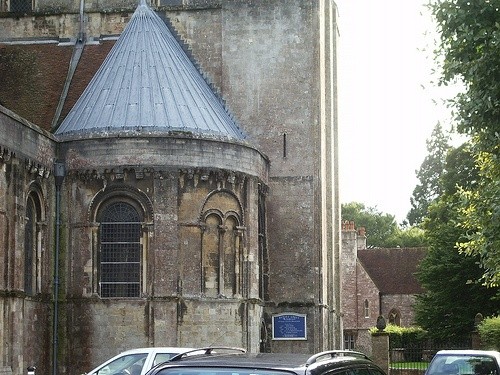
[80,347,216,375]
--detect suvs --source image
[143,344,385,375]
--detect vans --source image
[424,349,500,375]
